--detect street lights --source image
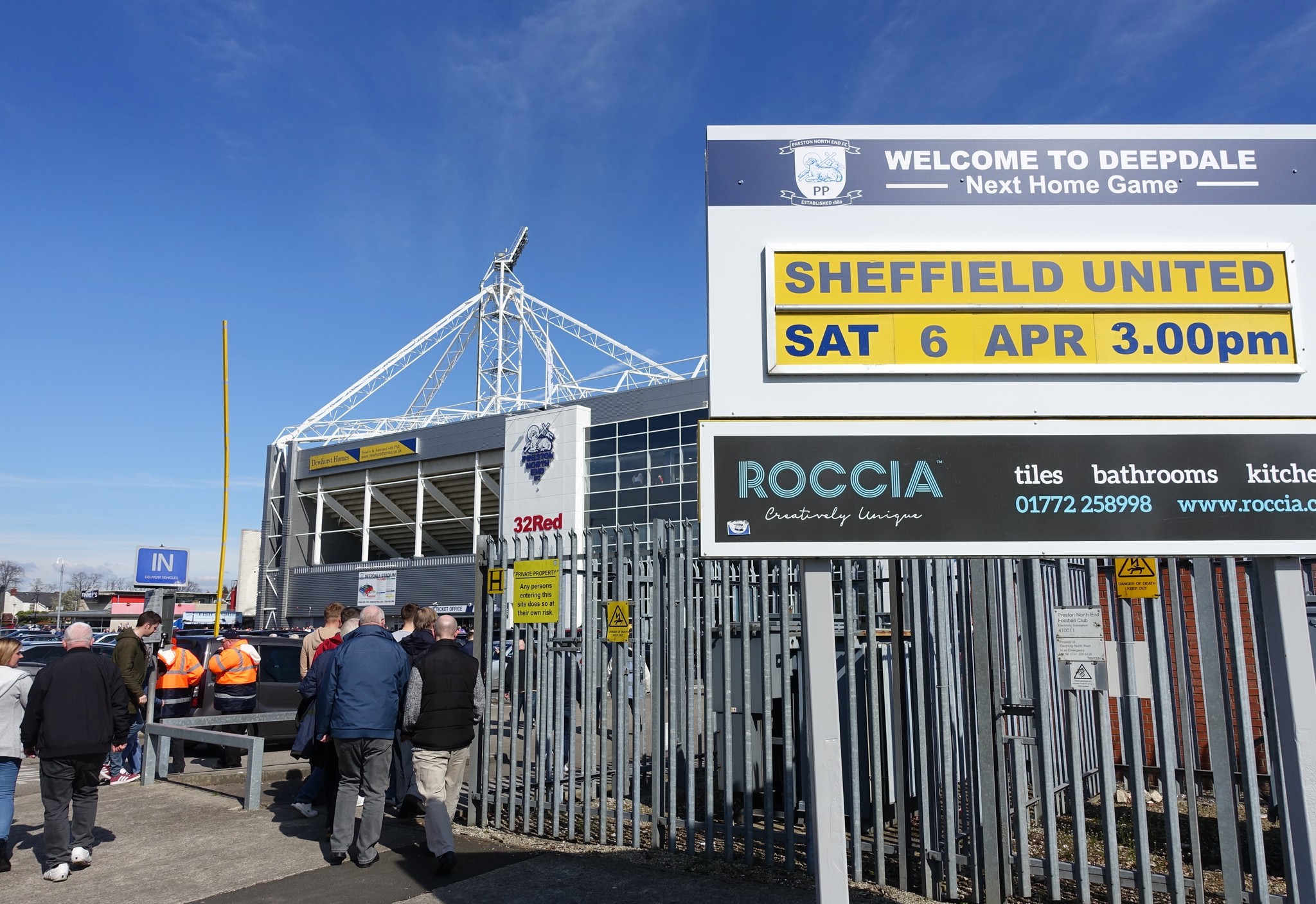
[56,557,65,631]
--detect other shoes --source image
[397,794,426,818]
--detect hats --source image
[216,629,241,640]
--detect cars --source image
[0,619,309,748]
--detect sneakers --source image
[100,765,111,779]
[110,768,141,785]
[71,847,92,866]
[325,827,332,841]
[356,794,365,807]
[209,763,230,769]
[290,803,318,817]
[44,863,70,882]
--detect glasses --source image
[221,640,232,645]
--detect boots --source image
[0,838,11,873]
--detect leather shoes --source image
[333,853,346,858]
[360,851,380,868]
[437,851,456,877]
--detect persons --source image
[100,609,162,786]
[400,614,487,879]
[207,628,262,769]
[155,631,204,774]
[203,625,322,640]
[314,605,412,868]
[0,636,34,874]
[13,624,66,637]
[97,622,129,643]
[119,657,168,776]
[19,621,131,882]
[290,599,651,820]
